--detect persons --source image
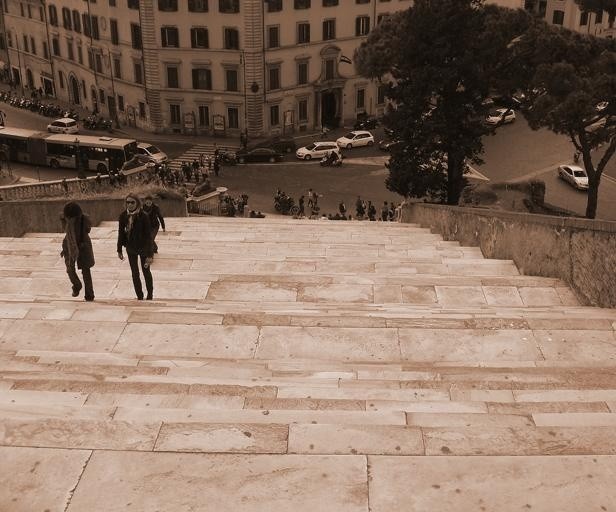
[321,123,329,139]
[30,86,50,100]
[155,142,231,186]
[140,195,166,253]
[298,189,397,222]
[57,202,94,302]
[329,149,342,167]
[9,78,16,92]
[116,192,158,302]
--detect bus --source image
[0,111,139,174]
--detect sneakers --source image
[138,294,153,300]
[85,295,94,300]
[72,288,81,296]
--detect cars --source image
[558,165,589,191]
[236,118,403,164]
[136,142,168,167]
[47,117,79,134]
[486,108,515,127]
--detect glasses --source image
[126,200,135,205]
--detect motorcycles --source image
[273,195,300,215]
[320,151,342,165]
[215,152,236,166]
